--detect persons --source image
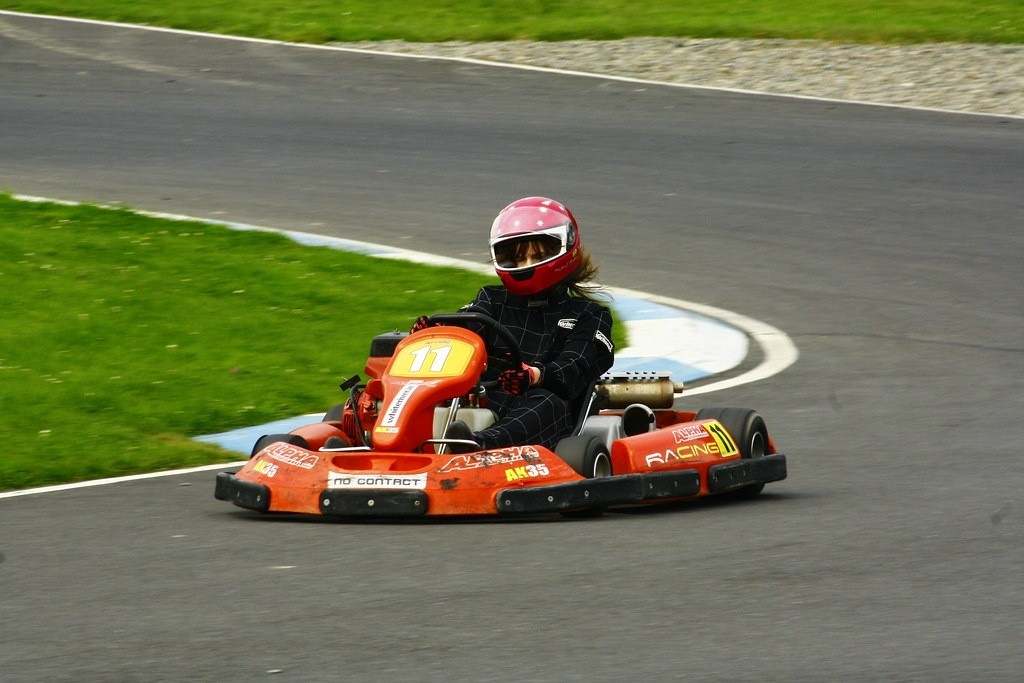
[409,196,615,453]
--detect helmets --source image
[489,197,581,297]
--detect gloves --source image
[498,353,535,395]
[410,315,441,335]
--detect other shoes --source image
[444,420,479,454]
[324,436,348,449]
